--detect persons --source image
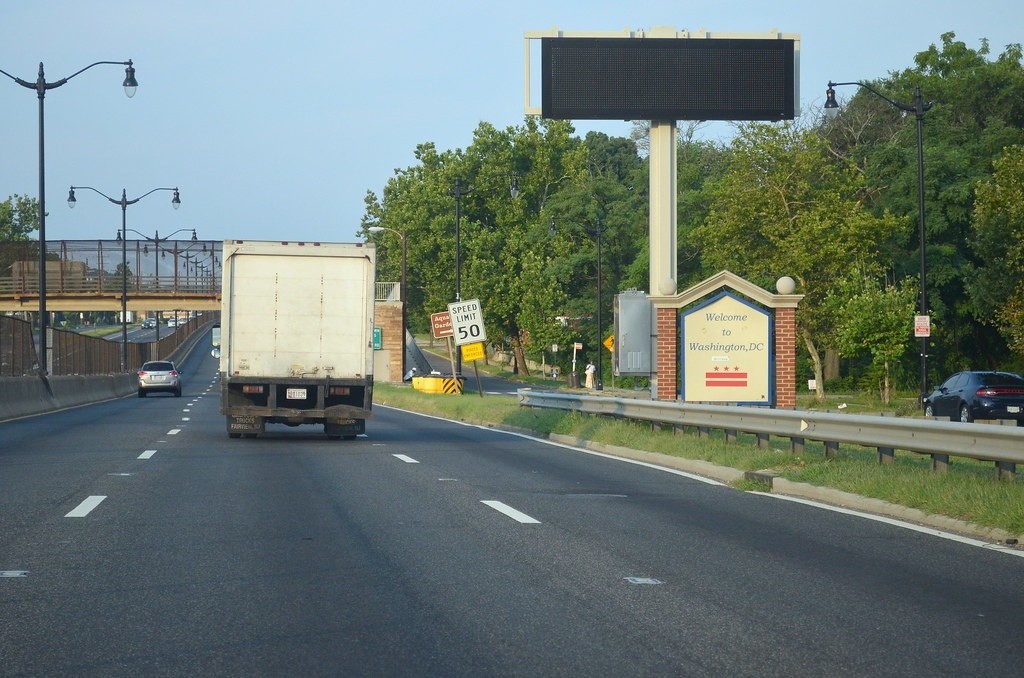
[584,361,596,388]
[404,367,420,381]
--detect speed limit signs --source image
[447,298,487,347]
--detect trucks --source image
[211,237,377,440]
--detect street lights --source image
[35,58,139,369]
[367,226,406,378]
[117,228,198,361]
[823,81,928,407]
[67,184,182,372]
[143,242,222,336]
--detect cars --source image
[141,320,153,329]
[924,371,1024,427]
[136,360,183,396]
[167,319,180,329]
[147,318,156,328]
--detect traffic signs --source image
[431,310,454,339]
